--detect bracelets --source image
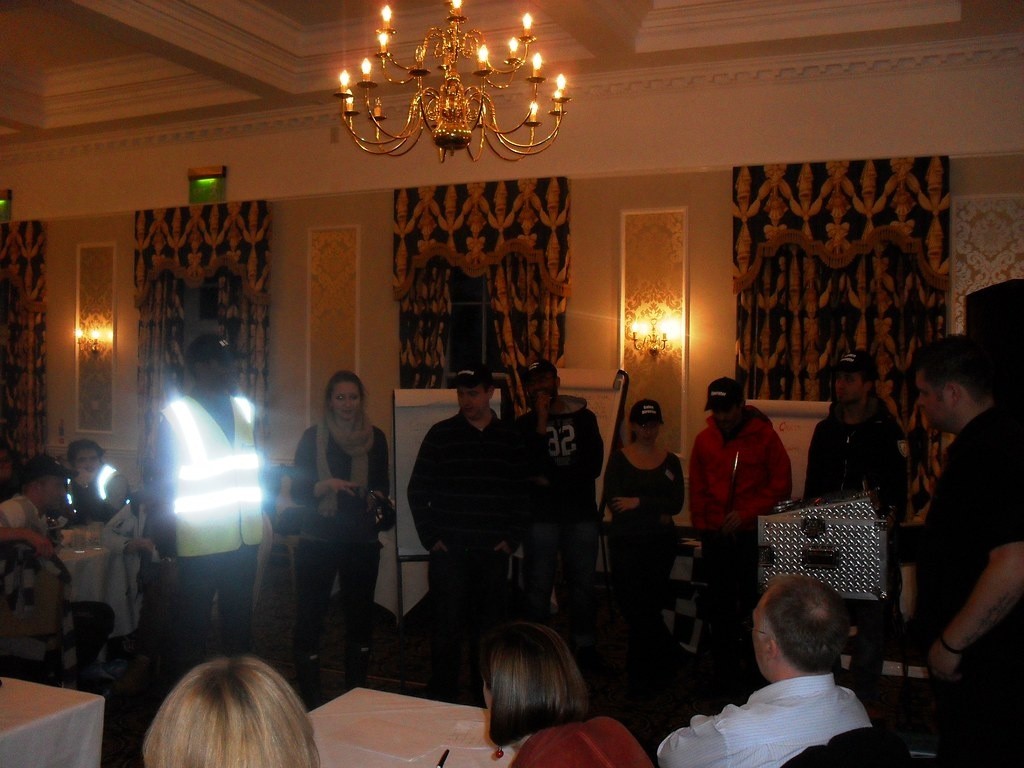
[940,635,963,654]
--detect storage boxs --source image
[758,488,898,598]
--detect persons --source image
[290,370,389,709]
[604,398,684,693]
[481,621,655,768]
[801,352,911,701]
[142,657,320,768]
[512,359,611,674]
[657,574,875,768]
[688,378,792,686]
[907,335,1024,768]
[141,334,279,668]
[0,439,177,692]
[407,363,531,705]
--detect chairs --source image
[0,537,93,689]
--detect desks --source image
[0,673,105,768]
[27,521,142,634]
[304,686,530,768]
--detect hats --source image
[526,358,558,381]
[447,363,493,389]
[630,398,664,426]
[831,350,876,374]
[186,333,245,362]
[23,452,78,480]
[704,376,743,411]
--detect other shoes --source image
[576,652,624,676]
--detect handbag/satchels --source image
[364,488,397,533]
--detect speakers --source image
[963,278,1024,420]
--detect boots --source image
[293,653,324,709]
[345,636,371,690]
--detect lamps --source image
[76,328,112,362]
[334,2,571,163]
[630,314,673,356]
